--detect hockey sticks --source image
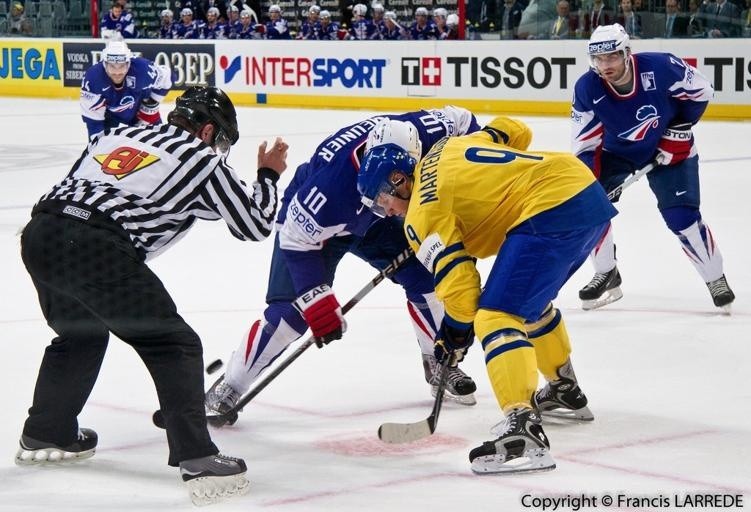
[378,365,451,444]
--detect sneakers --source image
[15,421,98,454]
[579,266,623,301]
[179,449,248,485]
[704,271,735,307]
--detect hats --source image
[14,3,24,12]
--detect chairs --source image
[1,1,107,37]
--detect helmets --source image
[101,42,131,84]
[357,117,424,218]
[587,23,632,83]
[168,83,240,162]
[161,3,459,27]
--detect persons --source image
[78,35,171,138]
[16,87,290,508]
[6,4,33,37]
[95,0,750,42]
[195,107,483,427]
[569,22,737,316]
[355,116,622,475]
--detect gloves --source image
[300,291,347,349]
[432,318,474,370]
[656,129,694,167]
[135,102,161,129]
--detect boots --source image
[535,379,595,413]
[423,359,477,396]
[468,408,557,463]
[204,372,240,426]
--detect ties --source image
[556,17,563,33]
[665,17,672,38]
[716,6,721,18]
[482,2,487,21]
